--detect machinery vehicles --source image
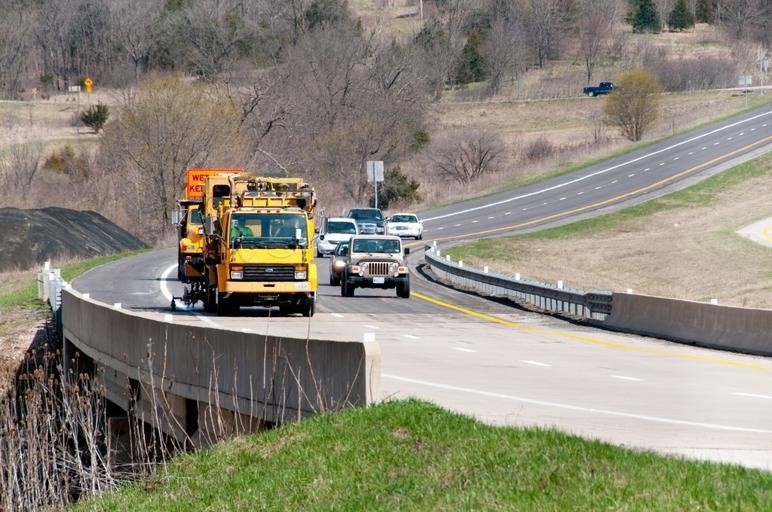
[171,169,316,317]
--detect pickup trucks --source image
[583,80,623,97]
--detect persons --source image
[231,215,253,238]
[382,241,394,252]
[274,218,296,237]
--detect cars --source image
[317,206,424,297]
